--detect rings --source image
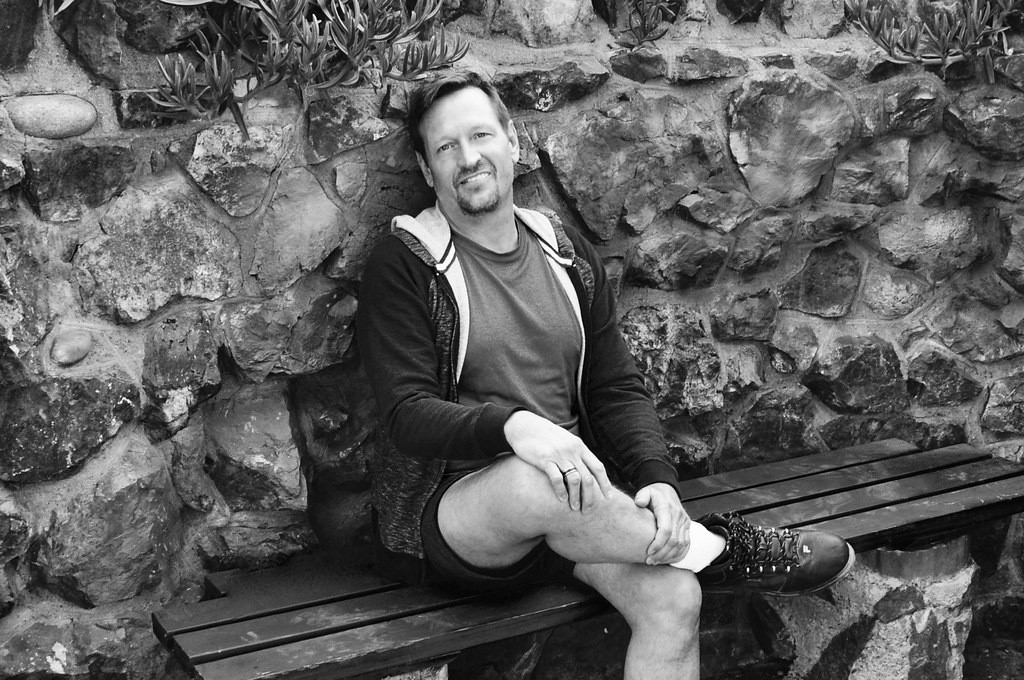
[562,468,576,476]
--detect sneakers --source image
[693,510,855,598]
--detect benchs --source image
[150,439,1024,680]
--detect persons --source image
[361,69,855,680]
